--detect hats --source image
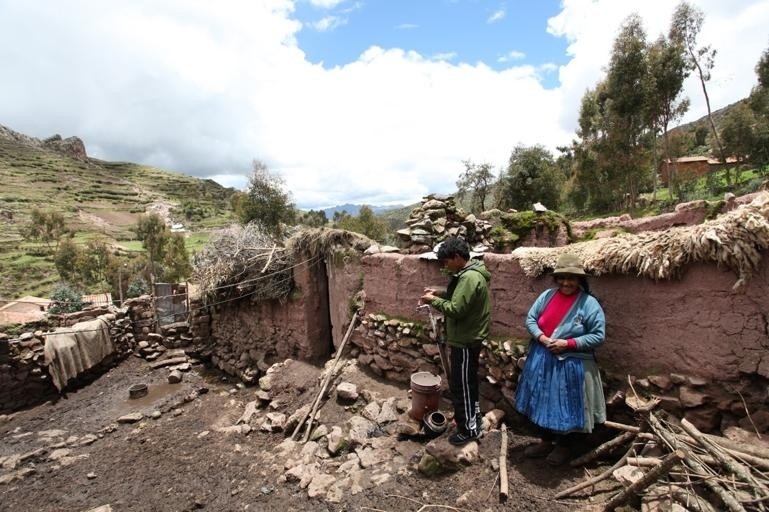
[549,254,591,279]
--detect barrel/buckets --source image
[409,371,442,419]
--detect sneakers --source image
[448,412,483,445]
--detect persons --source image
[507,254,606,466]
[422,236,491,446]
